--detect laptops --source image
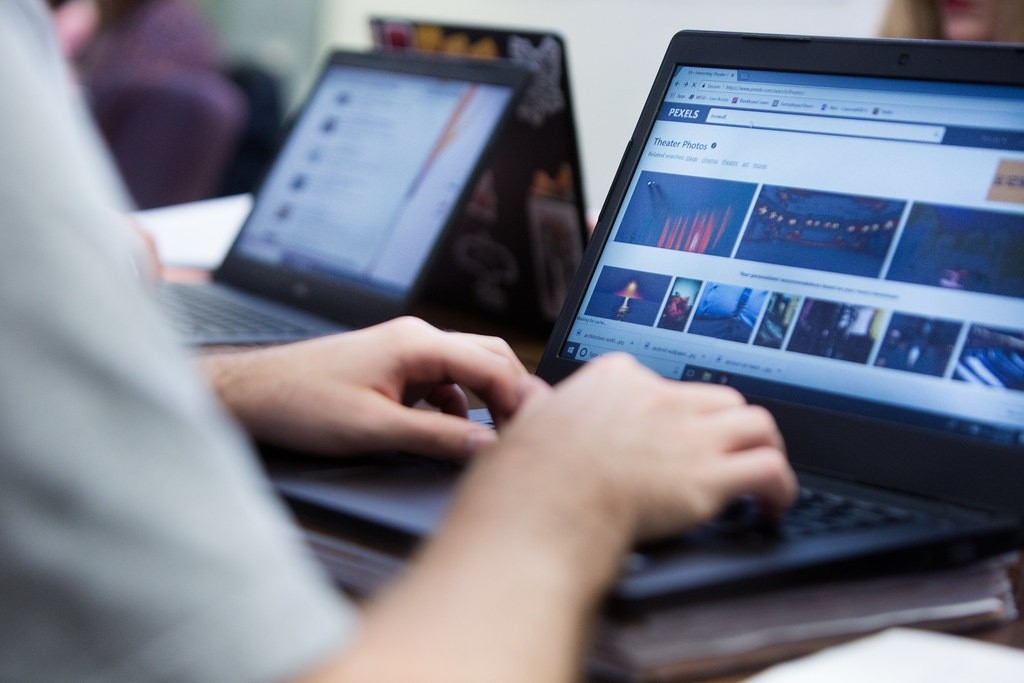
[368,17,591,348]
[164,46,536,352]
[256,28,1024,620]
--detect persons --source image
[0,0,798,683]
[880,0,1024,44]
[41,0,241,208]
